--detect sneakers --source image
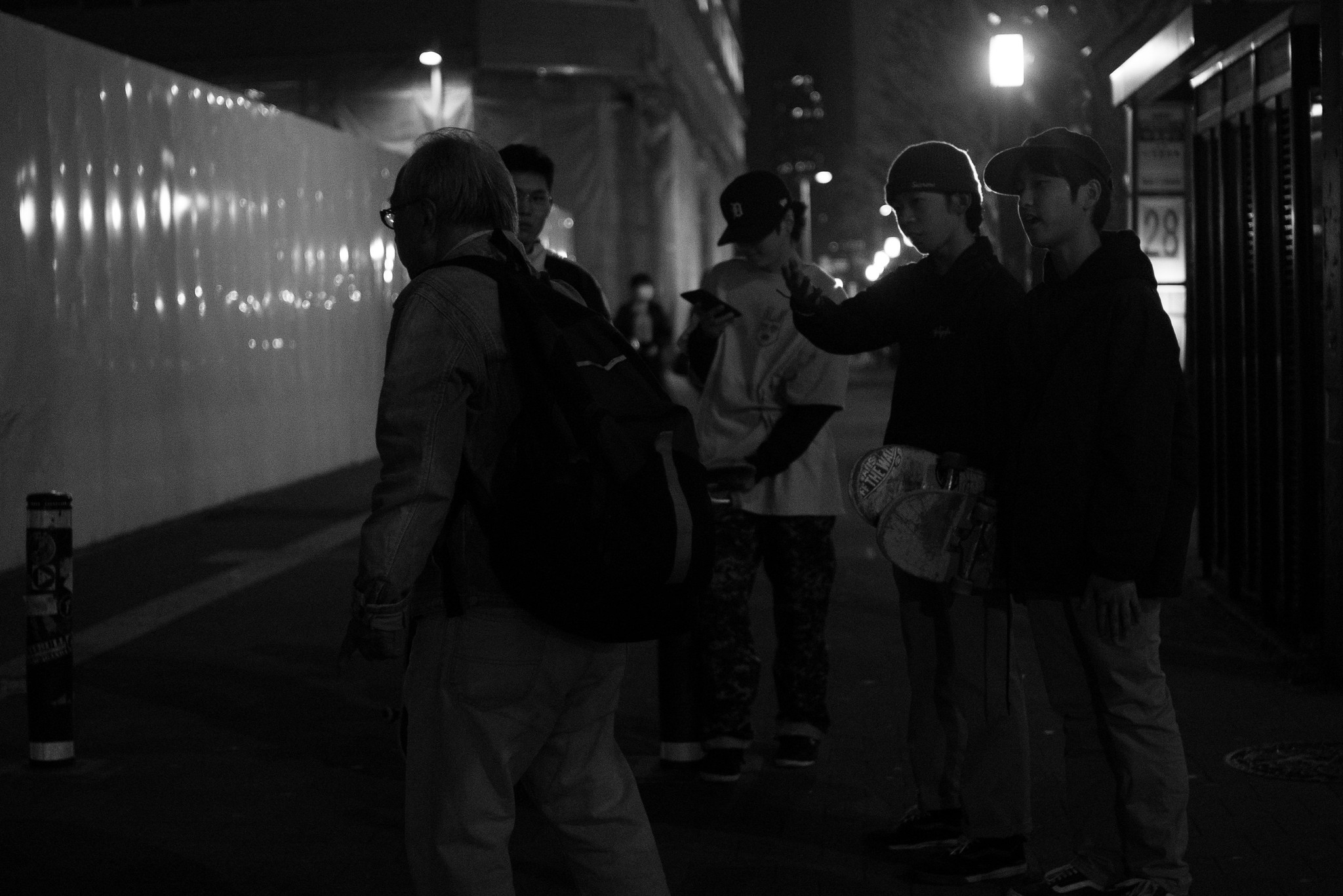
[1106,874,1176,896]
[888,805,967,850]
[1007,862,1110,896]
[911,835,1027,885]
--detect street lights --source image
[419,47,444,129]
[799,165,832,293]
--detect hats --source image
[983,128,1114,196]
[886,140,978,205]
[718,168,792,246]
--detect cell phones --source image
[680,289,742,322]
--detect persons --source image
[675,170,848,782]
[785,142,1032,882]
[615,273,673,378]
[341,128,667,896]
[499,143,610,321]
[982,128,1193,896]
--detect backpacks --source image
[421,229,711,647]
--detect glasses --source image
[378,192,422,231]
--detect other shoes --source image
[771,734,820,766]
[699,747,746,783]
[655,756,704,784]
